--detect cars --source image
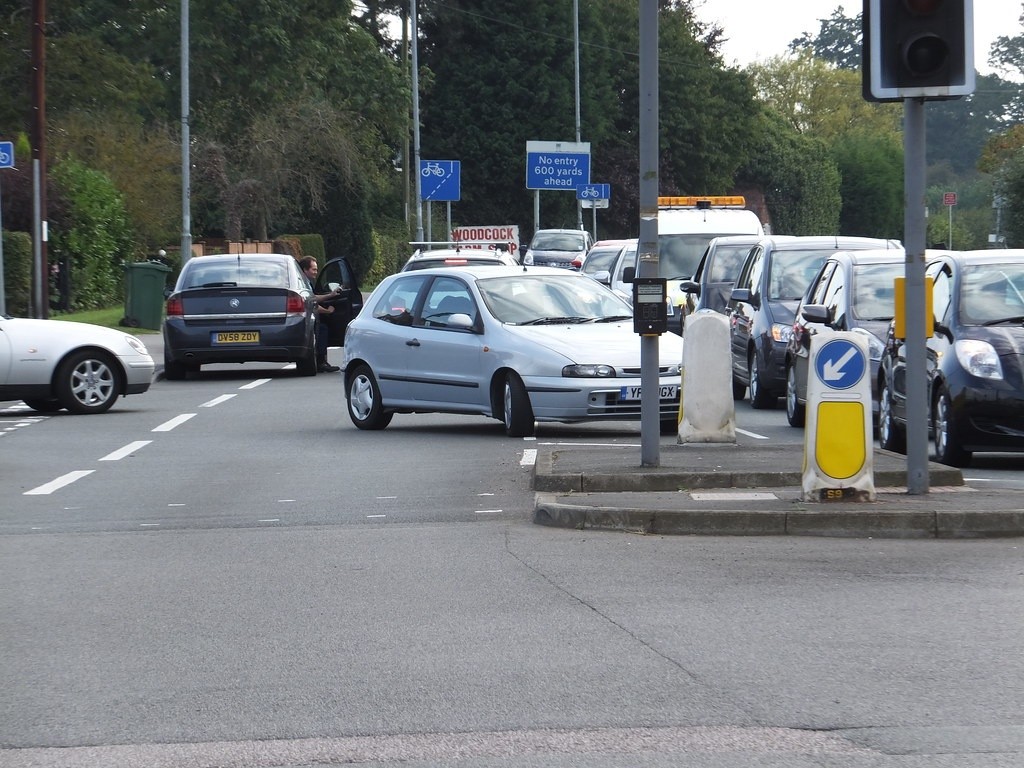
[582,193,1024,468]
[342,263,684,437]
[162,251,363,376]
[399,241,518,272]
[0,309,154,417]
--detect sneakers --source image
[317,362,340,373]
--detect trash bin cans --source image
[118,259,173,330]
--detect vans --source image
[519,229,594,272]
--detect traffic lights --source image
[862,1,976,105]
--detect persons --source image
[297,256,342,372]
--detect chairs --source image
[436,295,473,316]
